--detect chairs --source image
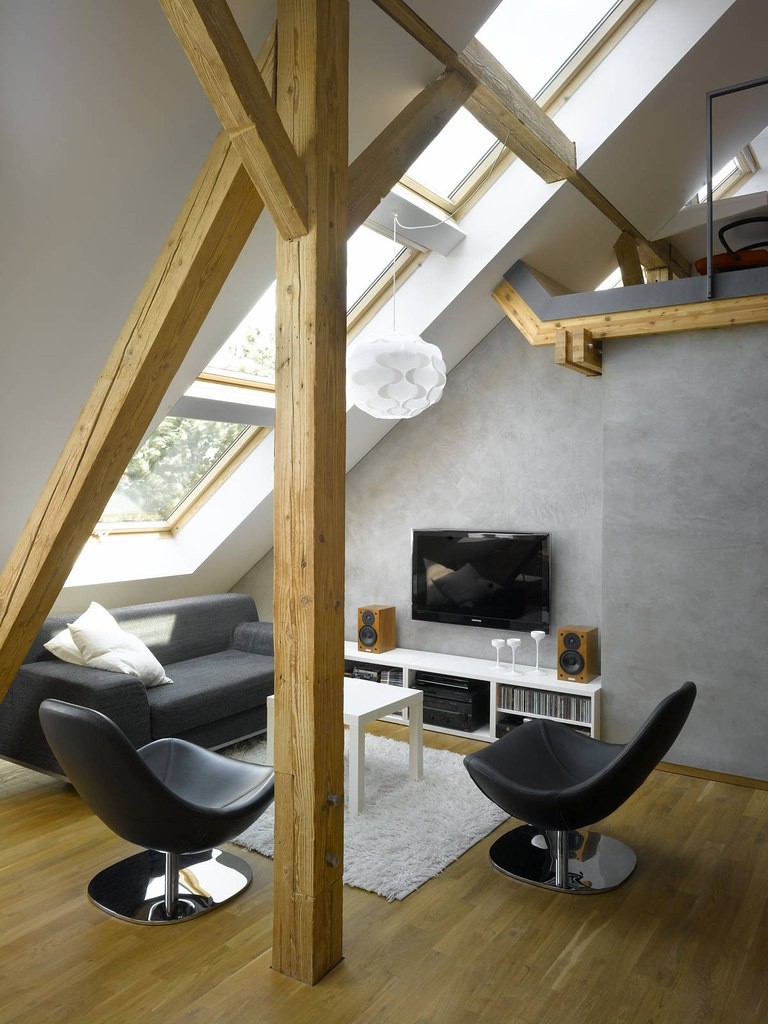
[695,216,768,276]
[39,699,274,926]
[464,681,698,895]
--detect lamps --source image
[346,213,447,420]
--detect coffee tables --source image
[266,676,424,817]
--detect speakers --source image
[358,605,396,653]
[557,625,597,683]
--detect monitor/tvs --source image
[412,530,551,636]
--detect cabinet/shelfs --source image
[344,641,601,744]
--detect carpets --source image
[217,728,510,903]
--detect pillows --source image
[43,601,174,687]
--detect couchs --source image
[0,593,274,784]
